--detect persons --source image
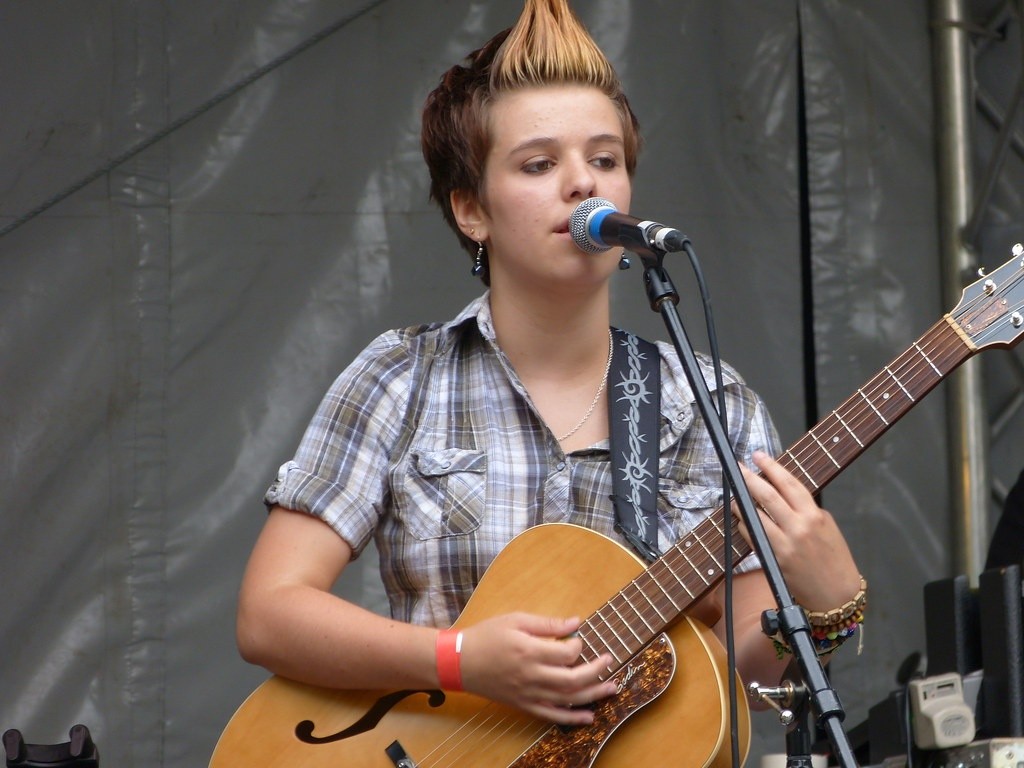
[236,0,868,768]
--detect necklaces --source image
[557,328,613,442]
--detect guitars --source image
[204,240,1024,768]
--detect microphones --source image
[568,197,692,256]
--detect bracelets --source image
[795,579,867,654]
[437,630,463,692]
